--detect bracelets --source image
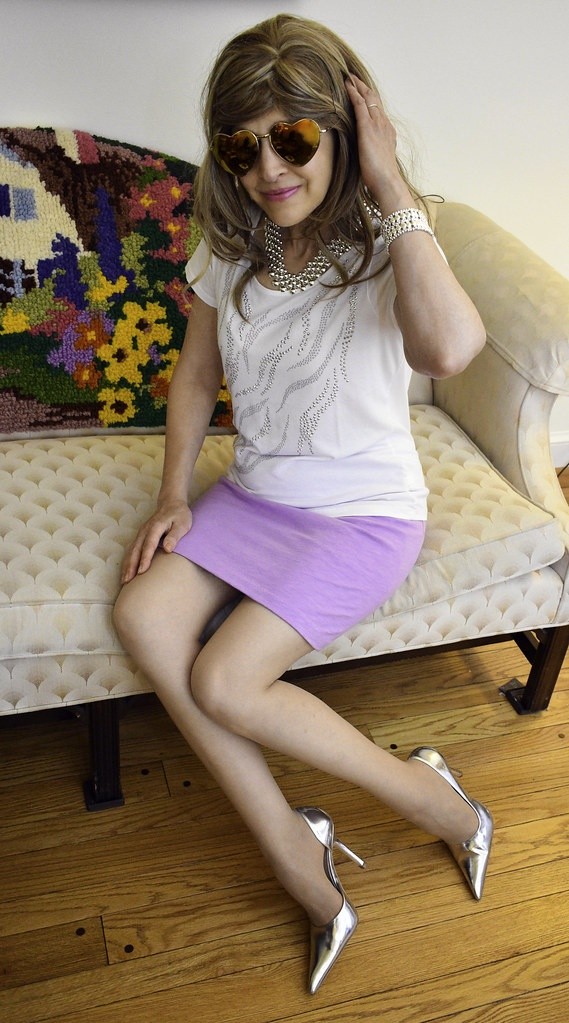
[380,209,436,256]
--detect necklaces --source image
[262,214,366,294]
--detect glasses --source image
[206,118,334,178]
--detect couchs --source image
[0,123,569,813]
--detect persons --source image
[113,14,495,995]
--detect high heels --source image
[295,806,367,995]
[407,746,493,900]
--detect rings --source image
[367,103,379,108]
